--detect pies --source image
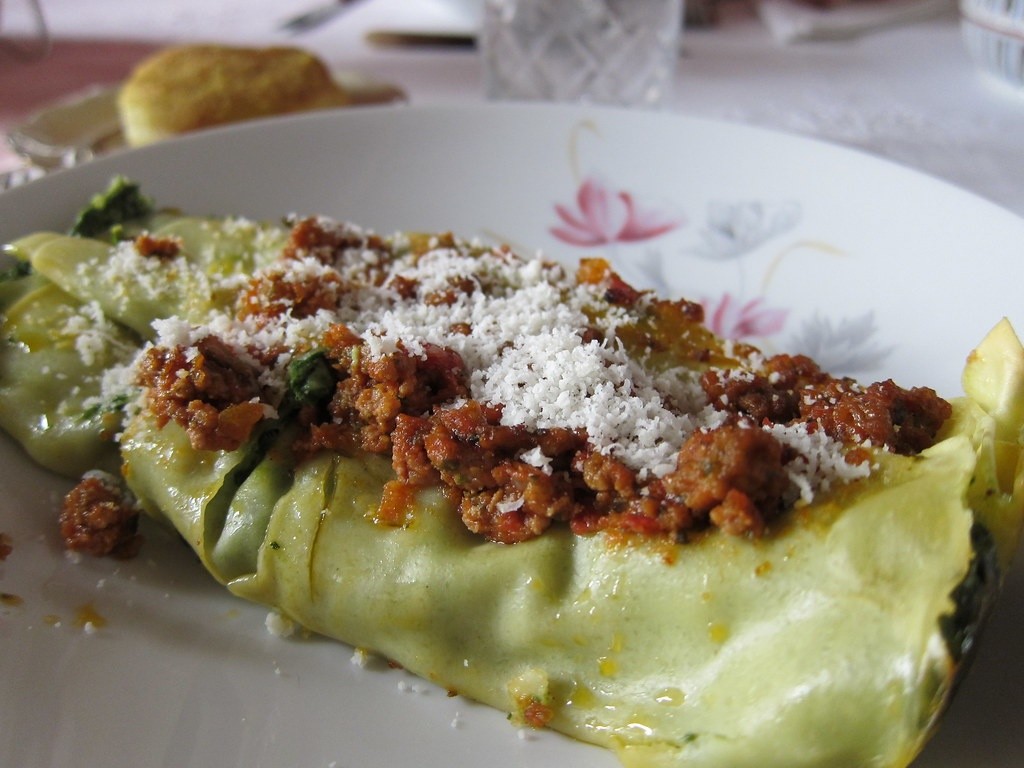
[1,208,1022,768]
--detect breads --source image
[119,48,334,148]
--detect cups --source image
[480,0,681,108]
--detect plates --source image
[0,100,1024,768]
